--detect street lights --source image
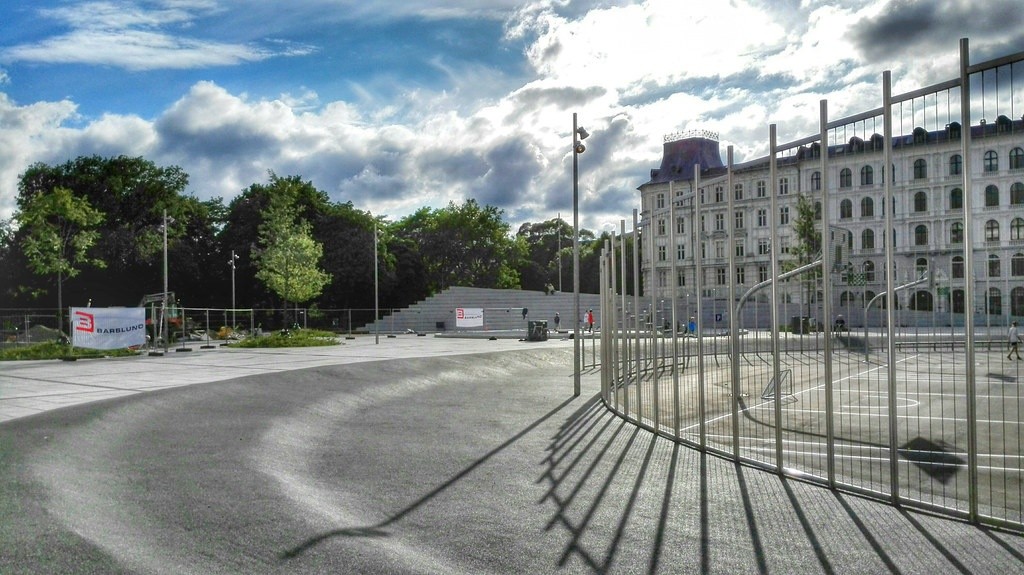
[228,255,240,330]
[158,215,175,354]
[374,229,385,344]
[574,127,589,397]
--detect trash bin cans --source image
[791,316,810,334]
[527,319,548,341]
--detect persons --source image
[836,315,845,332]
[543,283,557,296]
[1007,322,1022,360]
[689,317,696,334]
[554,312,560,332]
[583,309,595,333]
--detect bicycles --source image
[526,322,550,341]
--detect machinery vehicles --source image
[138,291,188,346]
